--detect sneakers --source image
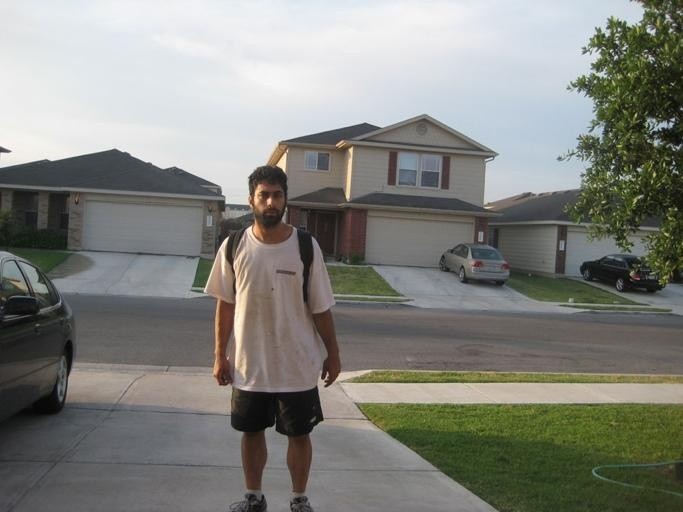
[287,495,313,512]
[224,492,268,512]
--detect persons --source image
[202,165,342,511]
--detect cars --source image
[0,250,77,423]
[440,242,510,285]
[580,254,667,292]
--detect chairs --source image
[473,252,480,258]
